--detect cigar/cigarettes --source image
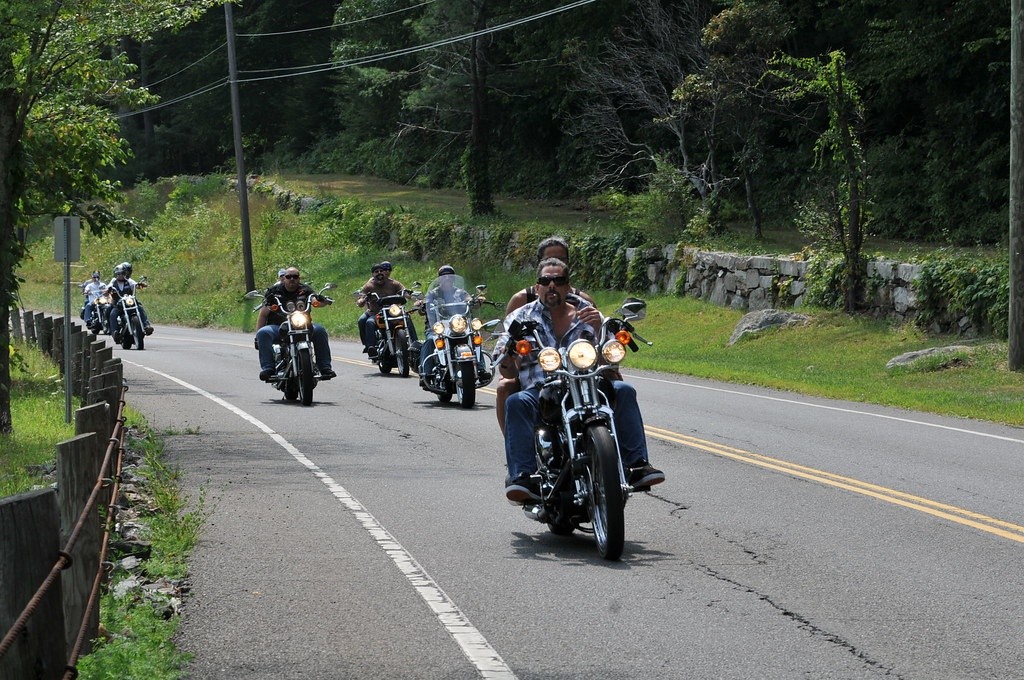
[553,294,557,296]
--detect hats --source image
[438,265,456,276]
[278,269,286,277]
[120,261,132,271]
[113,265,126,274]
[380,261,392,271]
[371,264,385,273]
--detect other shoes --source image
[320,369,336,378]
[363,346,368,353]
[113,331,121,345]
[145,325,154,335]
[368,346,378,361]
[478,373,492,382]
[259,369,276,381]
[86,319,91,327]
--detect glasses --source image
[536,276,570,287]
[93,276,99,278]
[285,275,299,279]
[374,270,383,273]
[280,275,284,278]
[117,272,125,275]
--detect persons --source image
[494,237,666,505]
[357,262,418,362]
[414,265,491,390]
[105,261,154,341]
[255,267,337,380]
[79,270,107,326]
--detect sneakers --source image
[629,459,666,488]
[505,472,541,506]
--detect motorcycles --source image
[404,284,495,409]
[79,290,113,335]
[102,275,152,350]
[352,281,423,377]
[241,282,338,407]
[483,296,651,563]
